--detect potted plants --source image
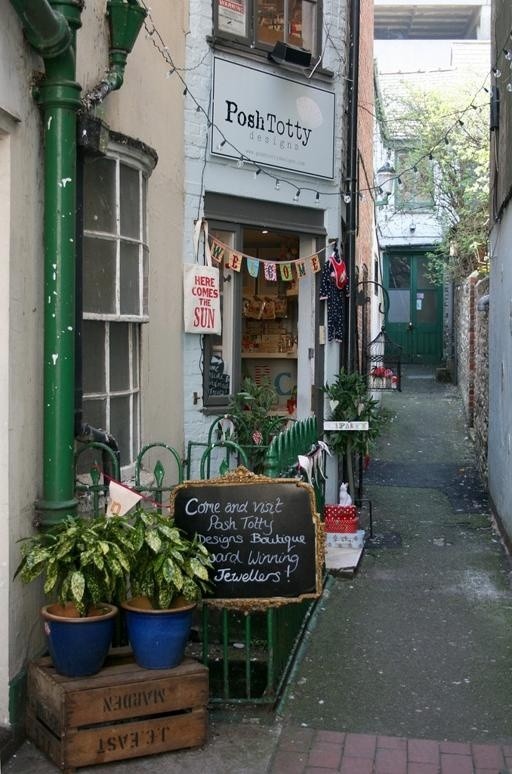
[121,509,214,670]
[13,516,137,677]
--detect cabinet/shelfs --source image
[243,248,299,421]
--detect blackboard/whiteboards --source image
[169,465,327,609]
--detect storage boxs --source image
[324,504,357,520]
[327,531,366,549]
[23,646,210,773]
[325,518,358,532]
